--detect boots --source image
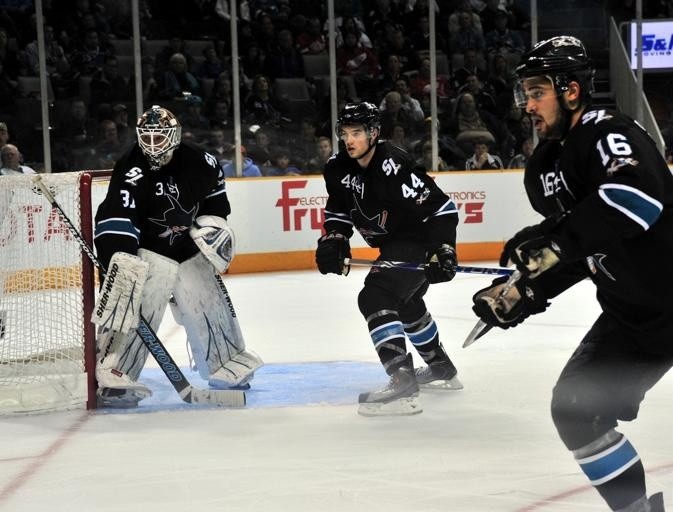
[414,342,457,384]
[359,353,419,403]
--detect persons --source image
[472,36,673,512]
[317,103,459,404]
[0,0,673,178]
[91,105,262,408]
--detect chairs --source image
[10,36,518,153]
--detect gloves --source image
[316,231,347,276]
[425,244,457,283]
[472,211,570,330]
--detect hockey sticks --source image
[33,175,246,408]
[462,265,520,348]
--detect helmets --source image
[513,36,594,109]
[136,105,182,158]
[335,101,381,137]
